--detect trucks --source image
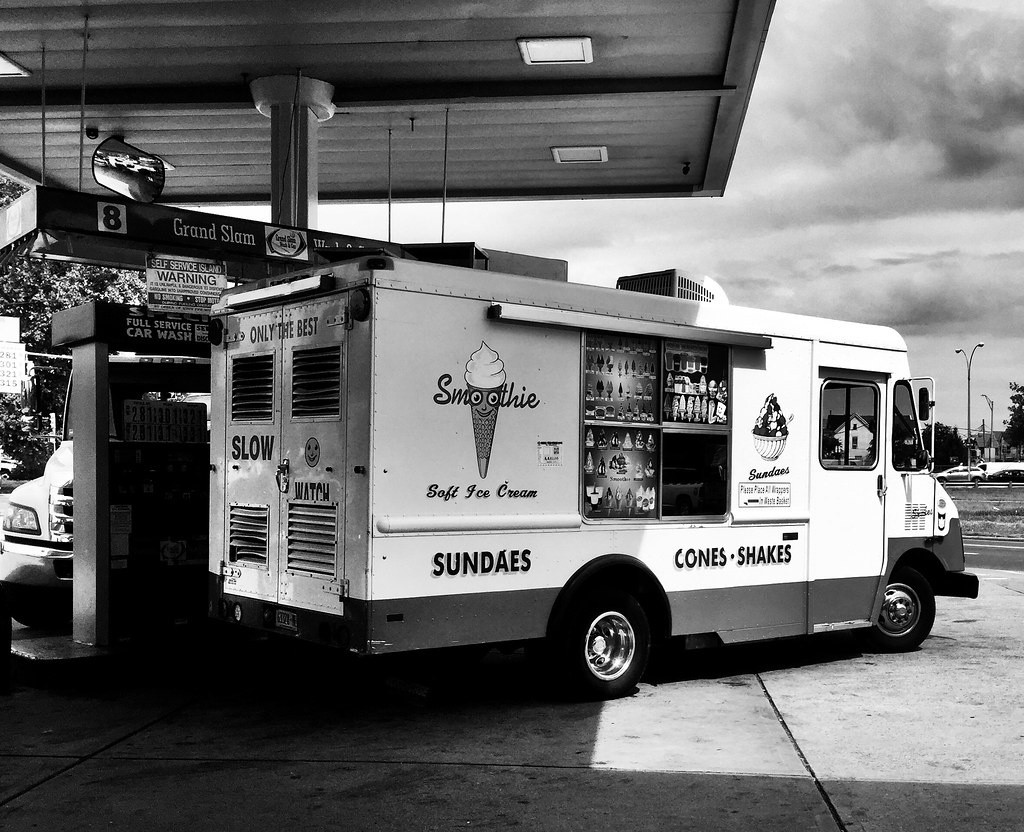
[204,250,980,700]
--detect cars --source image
[935,466,986,487]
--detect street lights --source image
[954,341,984,481]
